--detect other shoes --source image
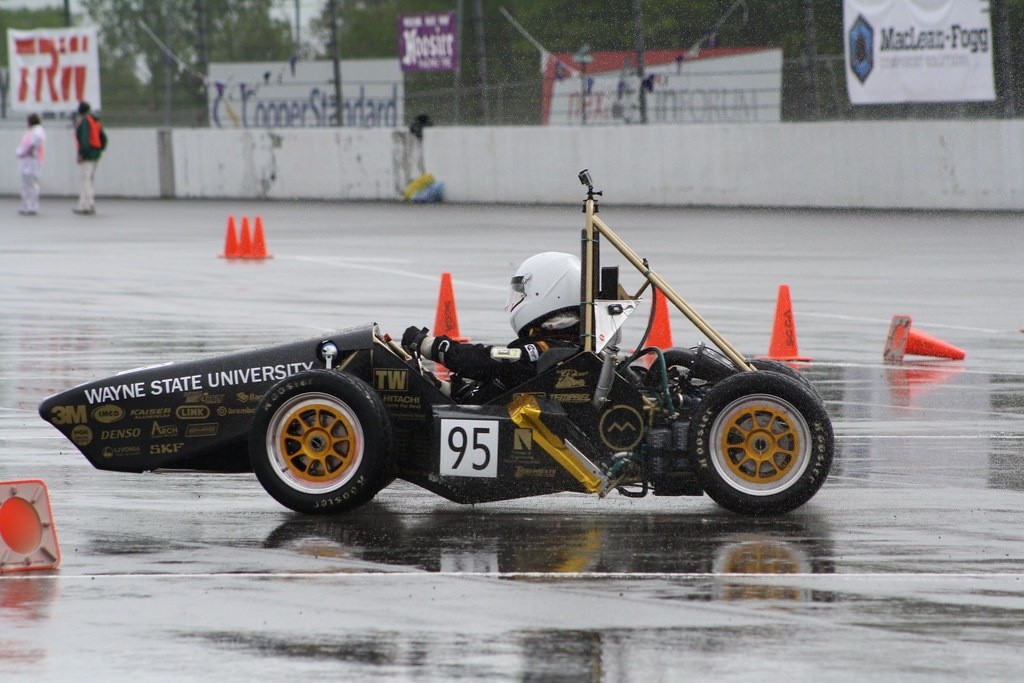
[19,208,36,215]
[73,208,93,214]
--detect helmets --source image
[504,252,581,337]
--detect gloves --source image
[401,325,429,354]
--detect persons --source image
[401,252,581,404]
[73,101,107,215]
[15,114,45,215]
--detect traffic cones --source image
[0,480,62,575]
[217,217,239,258]
[238,217,254,258]
[755,286,811,360]
[632,286,673,353]
[428,274,470,343]
[882,313,966,362]
[252,218,272,260]
